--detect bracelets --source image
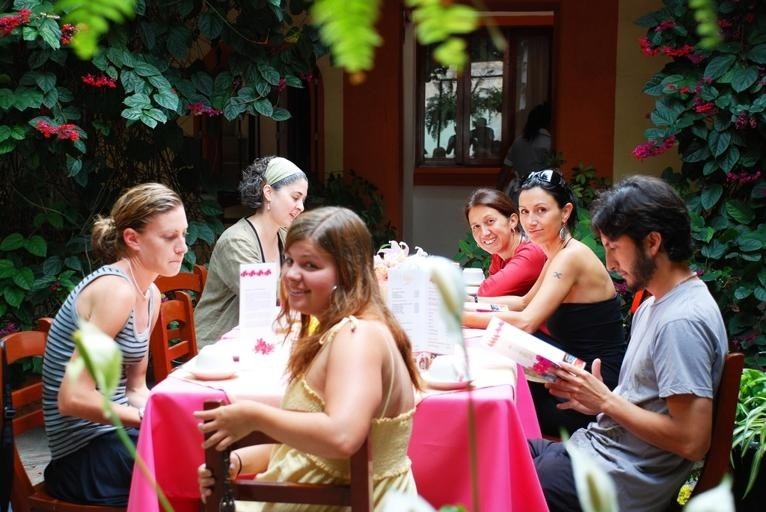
[234,450,243,475]
[474,295,478,304]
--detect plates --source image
[428,380,474,390]
[191,369,233,381]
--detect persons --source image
[193,206,435,512]
[446,125,457,159]
[466,190,545,297]
[193,157,309,351]
[468,118,494,159]
[433,148,446,158]
[525,174,728,511]
[495,104,562,192]
[41,182,188,506]
[460,169,625,436]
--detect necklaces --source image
[127,261,150,300]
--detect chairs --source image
[199,400,367,510]
[670,350,743,512]
[1,316,128,512]
[148,264,222,385]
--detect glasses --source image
[527,169,566,185]
